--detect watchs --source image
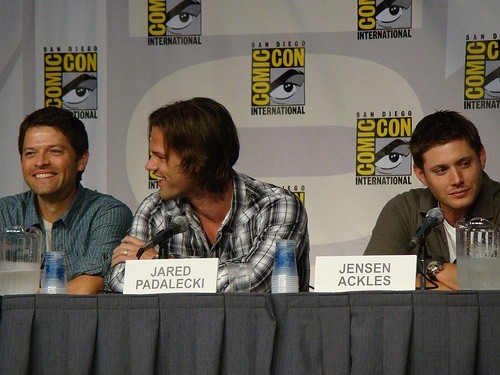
[428,261,446,276]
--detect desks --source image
[0,290,500,375]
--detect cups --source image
[271,238,300,293]
[226,263,252,292]
[40,251,68,294]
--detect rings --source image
[124,249,128,256]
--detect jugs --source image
[455,217,500,291]
[0,225,46,295]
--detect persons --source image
[108,97,310,292]
[0,106,133,294]
[365,108,500,292]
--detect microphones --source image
[138,215,191,257]
[406,205,444,250]
